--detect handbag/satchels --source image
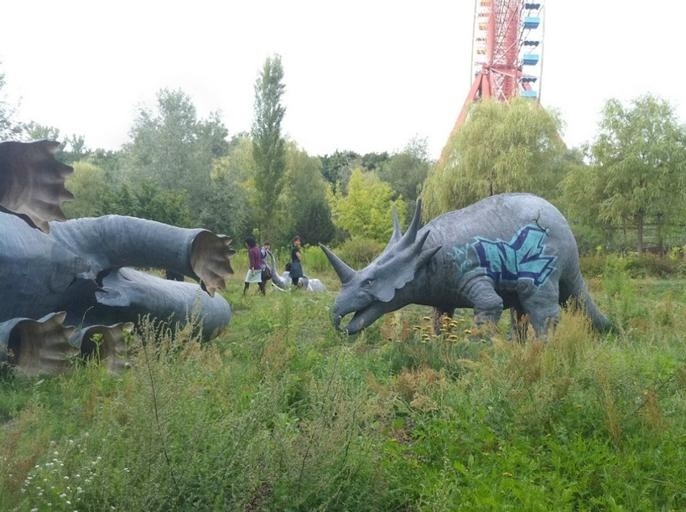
[262,266,272,281]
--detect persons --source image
[259,241,271,287]
[160,268,184,281]
[243,237,265,296]
[289,236,303,285]
